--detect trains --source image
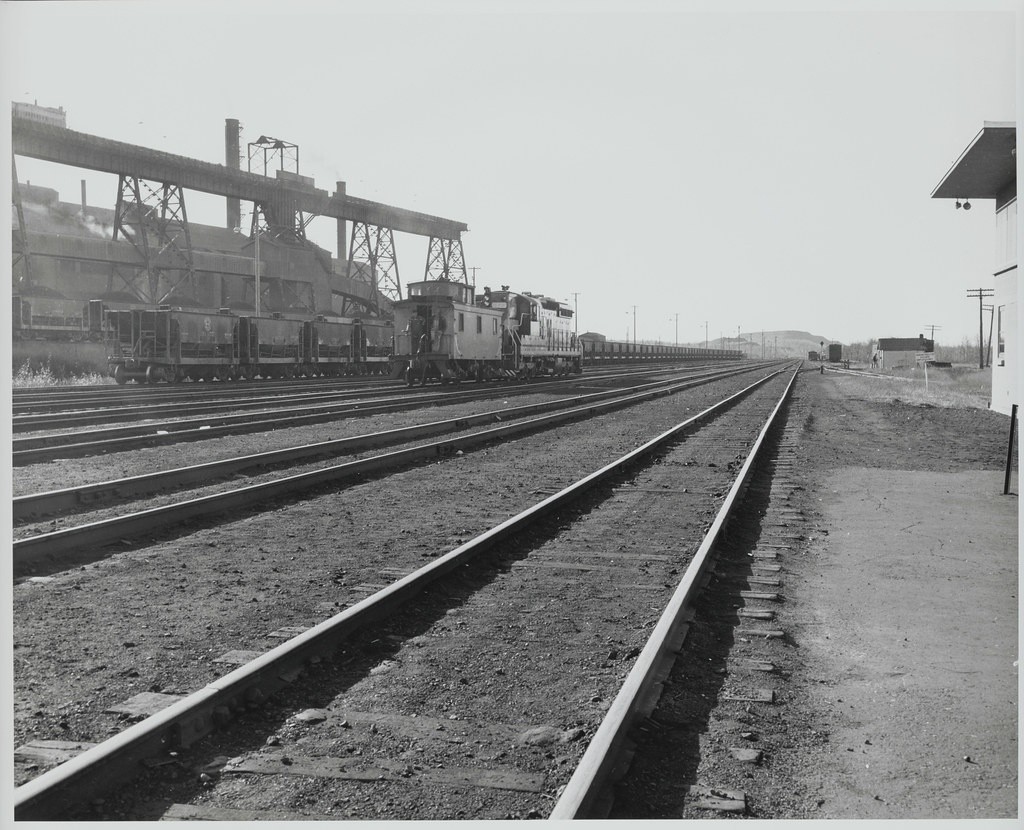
[807,351,817,361]
[105,276,587,386]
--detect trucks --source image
[823,344,841,362]
